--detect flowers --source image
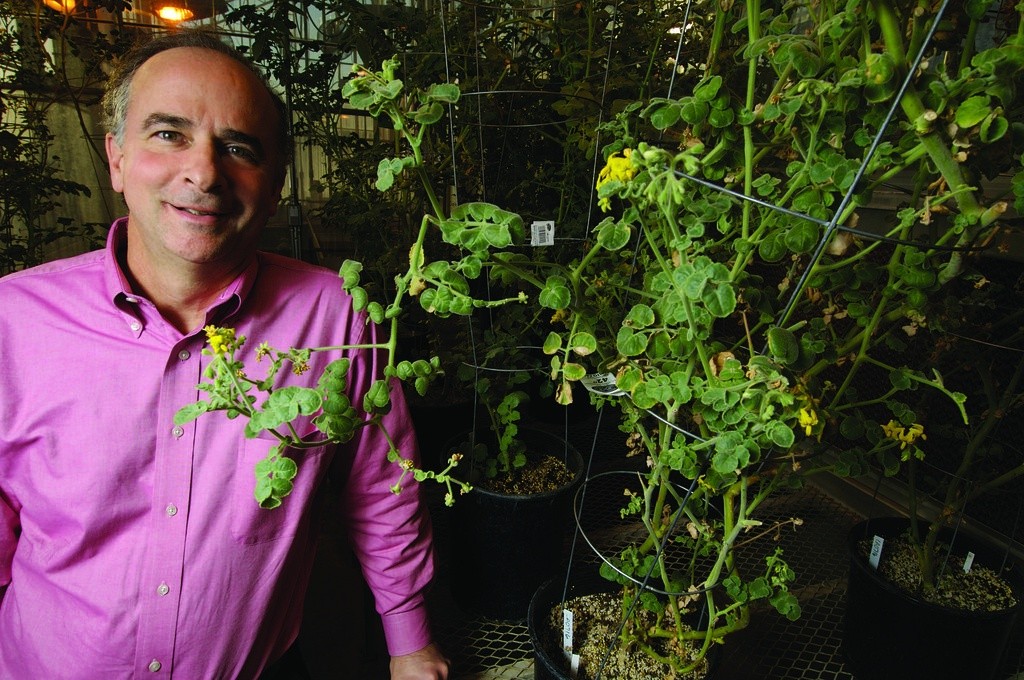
[174,0,1024,674]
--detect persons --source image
[0,33,452,680]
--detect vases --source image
[440,424,1024,680]
